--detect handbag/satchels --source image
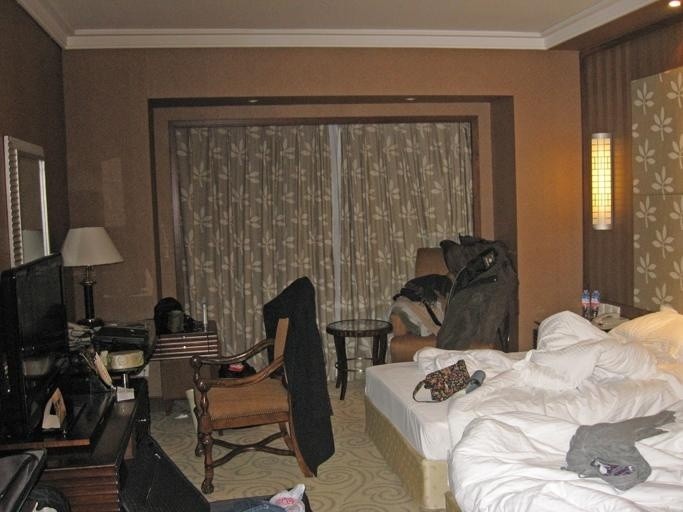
[424,360,470,402]
[401,274,452,302]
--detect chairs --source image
[388,247,511,365]
[188,306,319,497]
[117,433,311,512]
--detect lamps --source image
[588,131,614,232]
[57,221,126,330]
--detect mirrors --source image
[1,136,50,268]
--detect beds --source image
[442,455,681,511]
[362,305,682,512]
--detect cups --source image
[167,311,183,333]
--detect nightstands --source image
[530,313,629,351]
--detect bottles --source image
[590,289,601,318]
[580,289,591,321]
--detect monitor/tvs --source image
[0,251,89,442]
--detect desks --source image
[70,342,155,386]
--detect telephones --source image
[592,313,629,330]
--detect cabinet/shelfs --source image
[1,377,156,511]
[153,313,222,418]
